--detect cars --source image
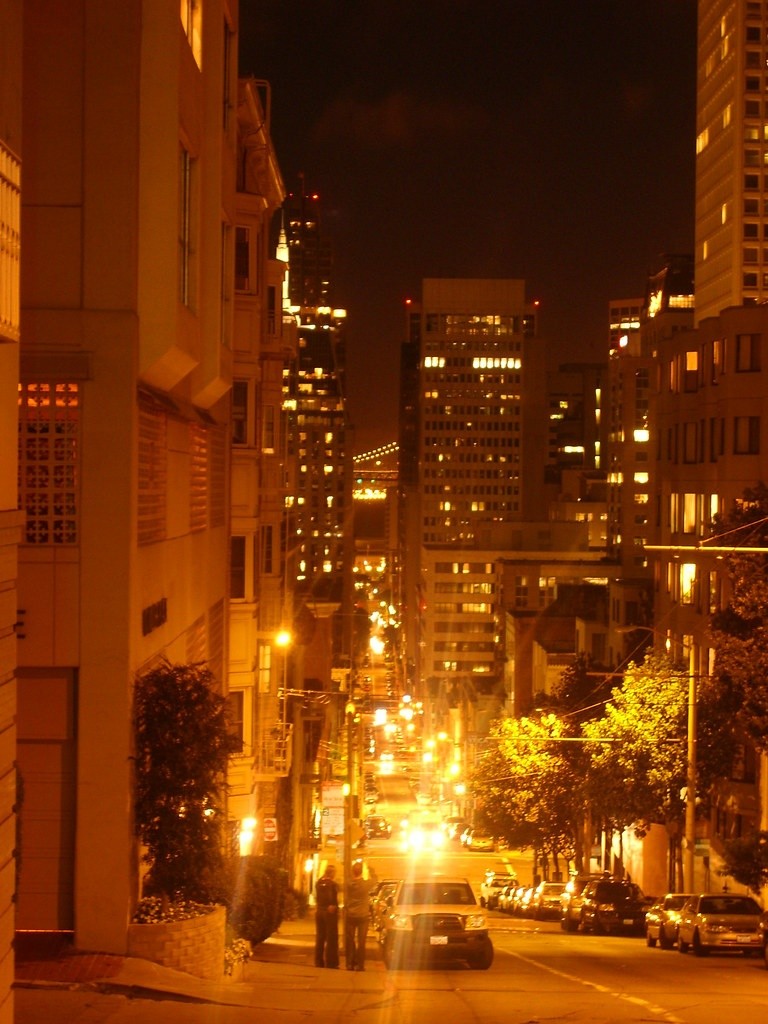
[645,893,767,958]
[440,816,495,850]
[364,814,394,840]
[478,871,648,936]
[366,877,494,970]
[364,771,381,802]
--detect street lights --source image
[614,624,700,893]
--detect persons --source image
[345,862,377,972]
[315,865,340,969]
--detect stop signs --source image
[263,819,278,840]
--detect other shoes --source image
[354,964,365,971]
[315,963,324,967]
[348,965,353,970]
[328,963,339,968]
